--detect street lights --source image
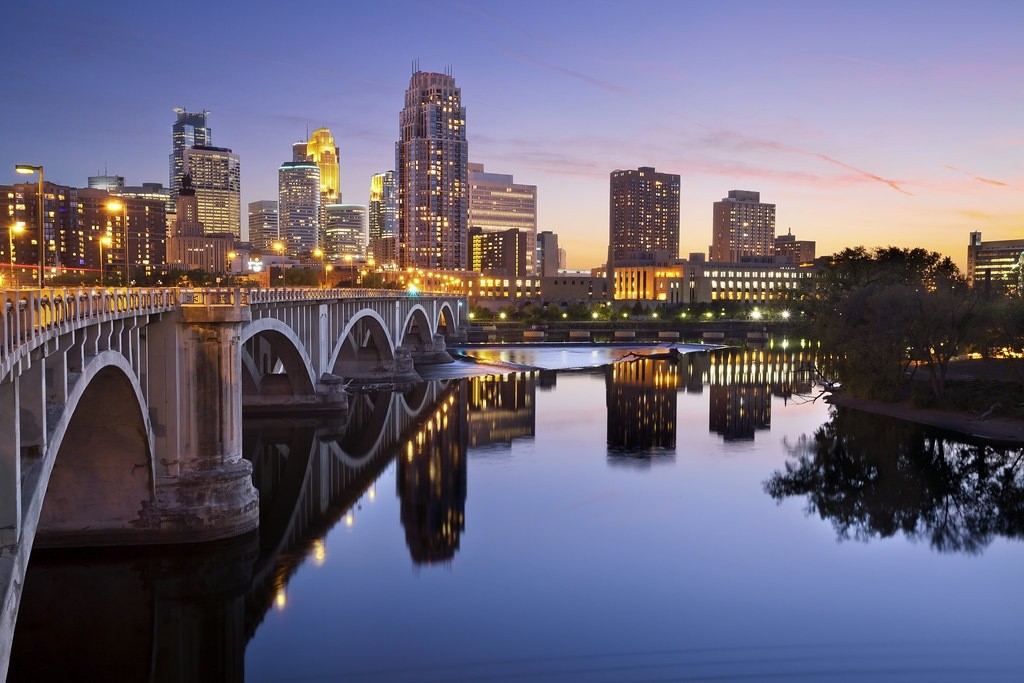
[312,248,376,288]
[400,267,462,294]
[107,201,131,289]
[99,237,111,288]
[227,253,236,289]
[16,163,45,288]
[274,242,285,289]
[8,224,23,287]
